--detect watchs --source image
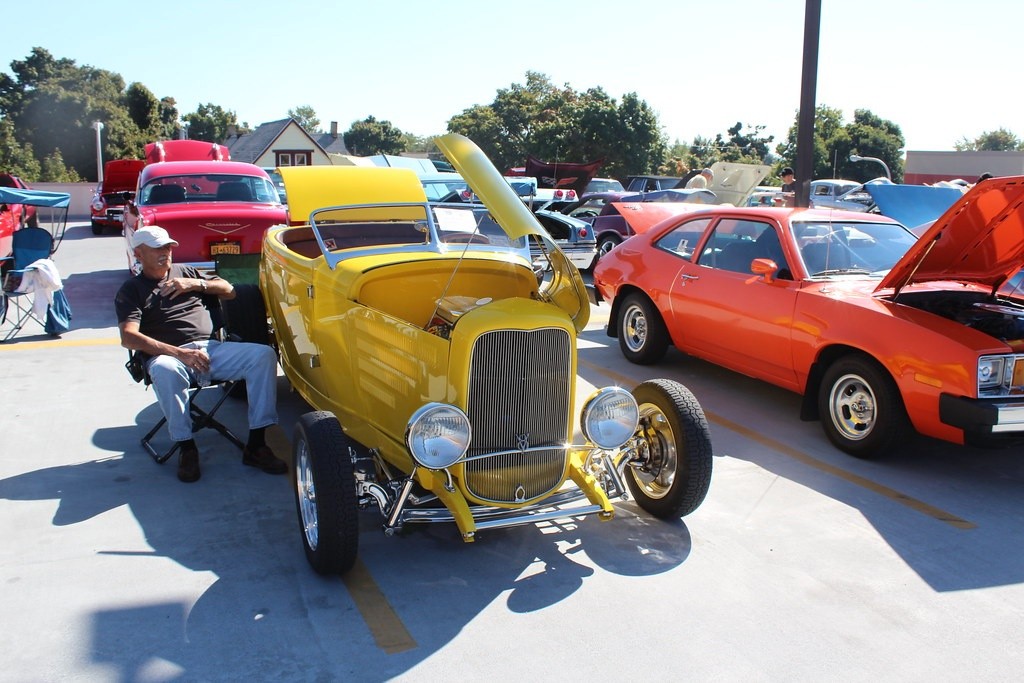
[198,277,208,294]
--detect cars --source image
[122,138,306,279]
[364,154,1024,294]
[88,159,147,237]
[240,164,289,205]
[0,171,41,275]
[218,131,714,578]
[590,175,1024,461]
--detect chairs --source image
[715,237,754,270]
[0,228,67,343]
[801,242,851,275]
[749,226,789,279]
[147,184,186,205]
[217,181,254,202]
[124,293,247,463]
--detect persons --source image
[112,225,291,484]
[684,168,715,191]
[780,168,815,209]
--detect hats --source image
[131,225,179,250]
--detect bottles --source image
[196,348,211,387]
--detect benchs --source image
[273,220,441,260]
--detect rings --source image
[194,363,198,367]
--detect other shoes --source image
[242,445,289,474]
[177,446,201,483]
